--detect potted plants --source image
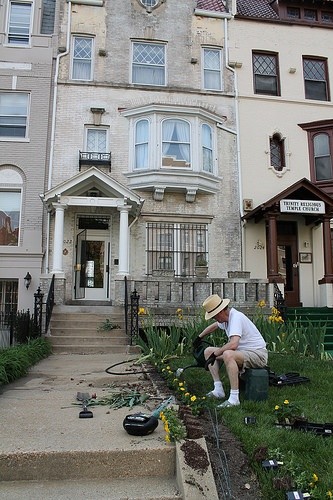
[194,259,208,278]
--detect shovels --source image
[77,392,93,418]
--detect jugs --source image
[175,339,224,378]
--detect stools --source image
[239,367,271,401]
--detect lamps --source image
[24,272,32,290]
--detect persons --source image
[193,294,267,409]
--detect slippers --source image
[207,391,217,400]
[218,401,233,408]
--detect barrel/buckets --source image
[244,366,270,400]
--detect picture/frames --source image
[300,252,312,263]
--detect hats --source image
[202,294,231,320]
